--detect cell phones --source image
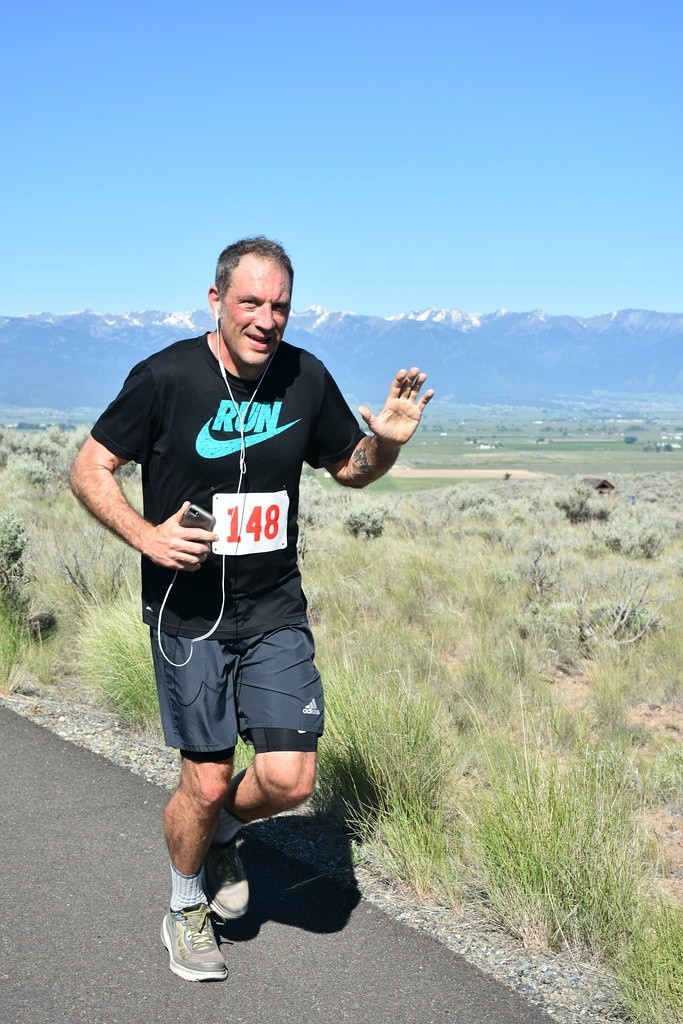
[179,504,216,544]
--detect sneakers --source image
[199,837,249,919]
[159,904,227,982]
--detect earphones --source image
[214,304,220,320]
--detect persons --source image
[70,237,435,982]
